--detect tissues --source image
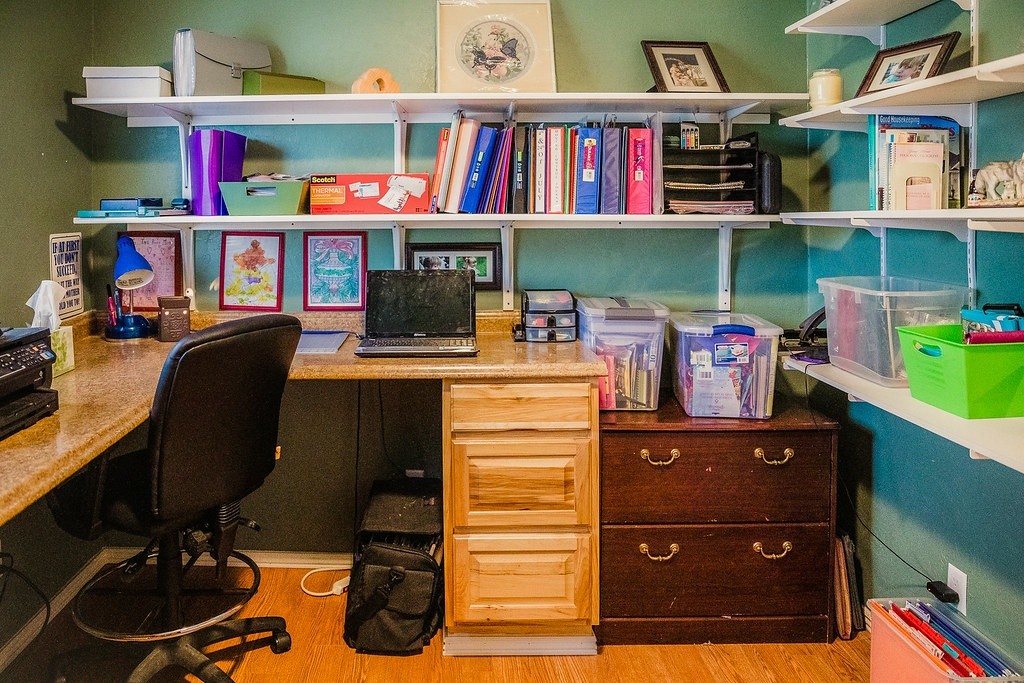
[25,280,76,379]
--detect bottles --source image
[810,69,844,109]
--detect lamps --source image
[105,235,154,340]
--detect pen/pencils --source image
[106,283,117,327]
[114,286,122,319]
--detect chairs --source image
[46,314,302,683]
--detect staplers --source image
[510,322,524,342]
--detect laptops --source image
[354,269,479,356]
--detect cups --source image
[156,296,191,341]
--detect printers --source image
[1,326,59,438]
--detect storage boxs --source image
[310,173,430,214]
[82,66,173,98]
[867,597,1024,683]
[218,181,310,215]
[895,324,1024,418]
[817,275,970,387]
[669,312,783,419]
[576,297,670,411]
[100,198,162,210]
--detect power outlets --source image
[946,563,967,616]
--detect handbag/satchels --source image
[171,27,272,96]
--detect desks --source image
[0,309,608,672]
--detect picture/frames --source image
[854,31,962,98]
[117,230,182,313]
[302,231,368,311]
[640,40,731,93]
[405,241,502,289]
[219,230,285,312]
[435,0,557,93]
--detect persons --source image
[423,256,441,269]
[462,257,479,276]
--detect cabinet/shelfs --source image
[0,0,1024,683]
